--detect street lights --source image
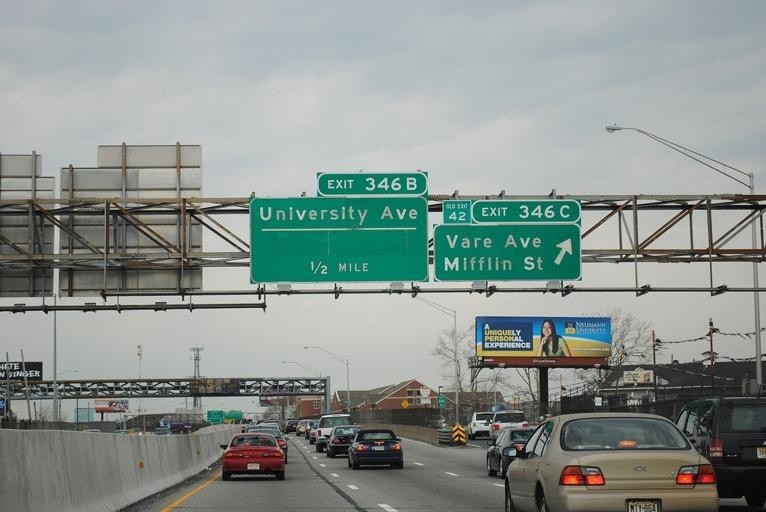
[137,344,144,415]
[606,126,766,386]
[58,370,78,377]
[282,347,350,412]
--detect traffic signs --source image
[251,172,428,282]
[433,199,582,280]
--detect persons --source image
[537,319,572,358]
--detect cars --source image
[83,422,193,434]
[220,414,403,480]
[467,396,766,512]
